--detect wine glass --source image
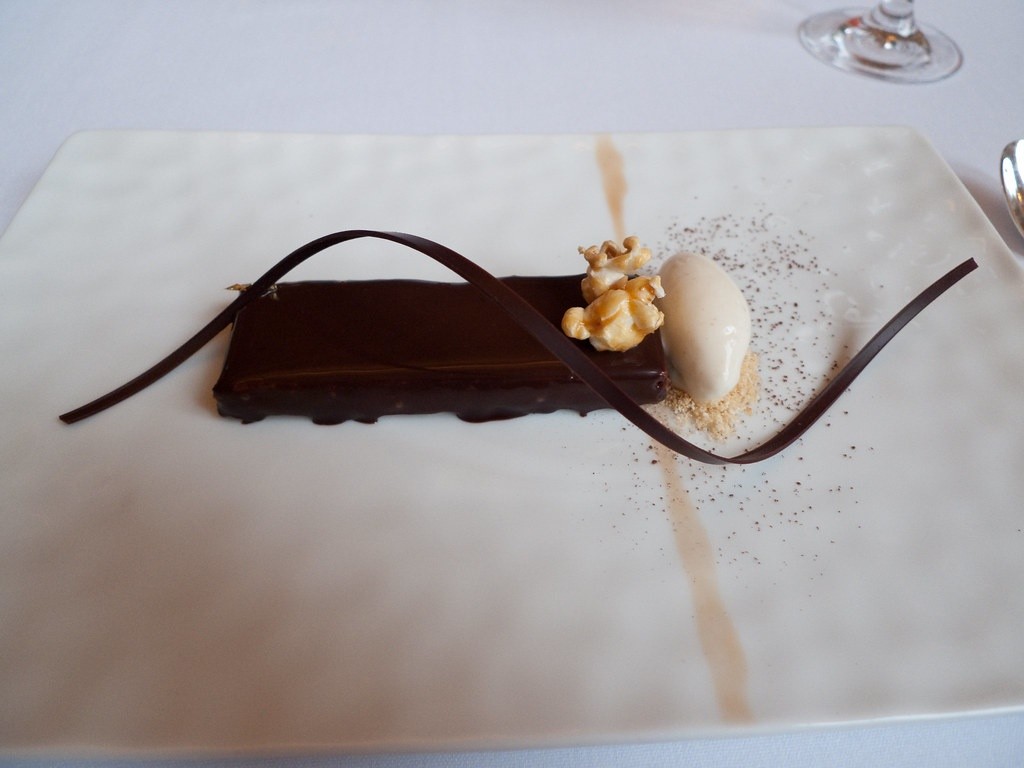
[799,1,960,83]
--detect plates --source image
[2,125,1024,755]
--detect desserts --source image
[59,229,979,467]
